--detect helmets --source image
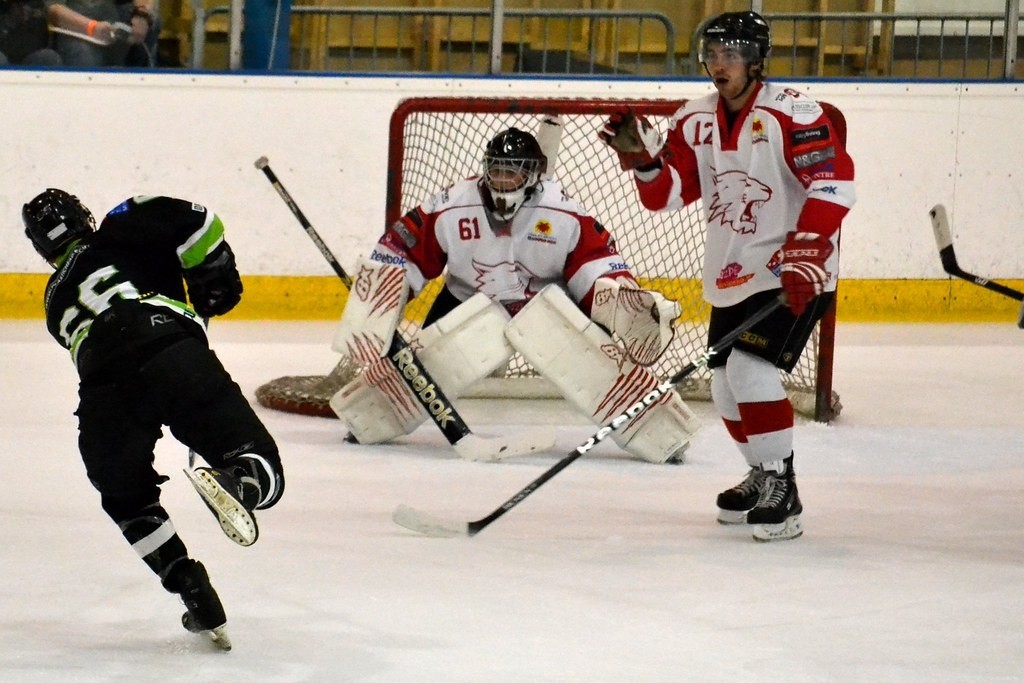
[692,10,772,64]
[483,127,547,221]
[22,188,96,270]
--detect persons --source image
[597,11,856,546]
[329,127,703,463]
[22,188,285,653]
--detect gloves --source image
[598,105,668,171]
[187,270,243,318]
[780,230,834,316]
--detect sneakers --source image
[746,449,804,543]
[183,457,262,547]
[715,464,763,526]
[181,561,232,651]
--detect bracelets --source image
[88,21,96,36]
[133,9,153,26]
[0,0,153,67]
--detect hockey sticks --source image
[185,287,222,469]
[927,201,1024,301]
[534,112,563,183]
[390,291,787,541]
[252,154,562,464]
